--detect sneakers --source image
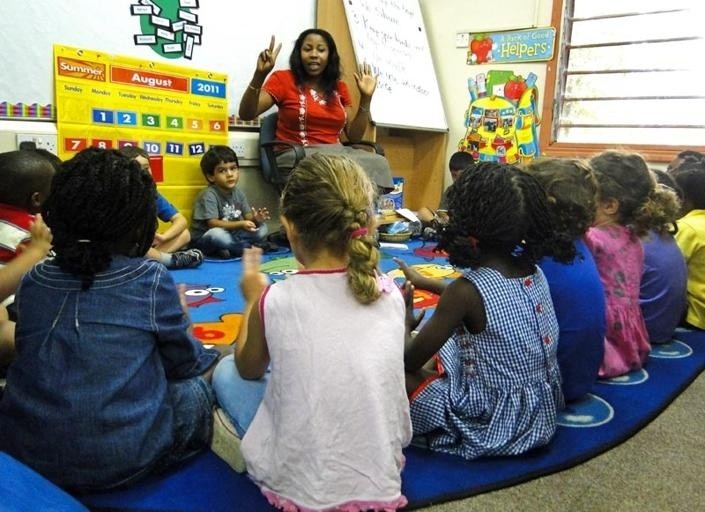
[171,249,202,267]
[212,405,247,473]
[217,250,230,259]
[424,227,441,240]
[258,241,278,252]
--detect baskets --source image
[380,232,412,242]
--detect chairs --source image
[257,113,384,252]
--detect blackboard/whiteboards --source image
[340,0,450,134]
[0,0,317,135]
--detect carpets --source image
[95,235,705,512]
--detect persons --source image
[237,28,395,221]
[204,153,415,511]
[418,150,474,240]
[582,149,705,375]
[392,156,607,404]
[394,158,577,460]
[189,146,279,259]
[0,146,231,495]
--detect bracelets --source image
[249,80,260,95]
[360,105,370,113]
